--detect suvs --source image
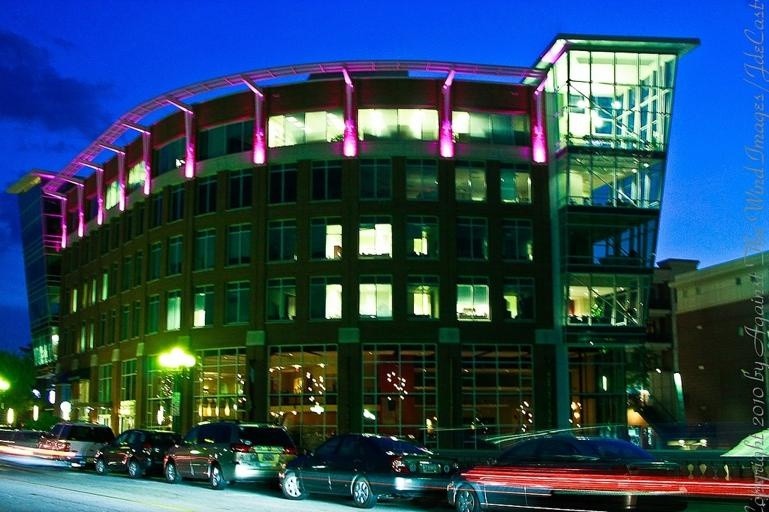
[35,420,116,472]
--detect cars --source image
[447,436,688,512]
[719,430,769,480]
[1,428,44,465]
[93,429,181,478]
[276,432,454,510]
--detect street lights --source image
[158,347,196,432]
[0,377,11,425]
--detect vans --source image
[162,418,299,490]
